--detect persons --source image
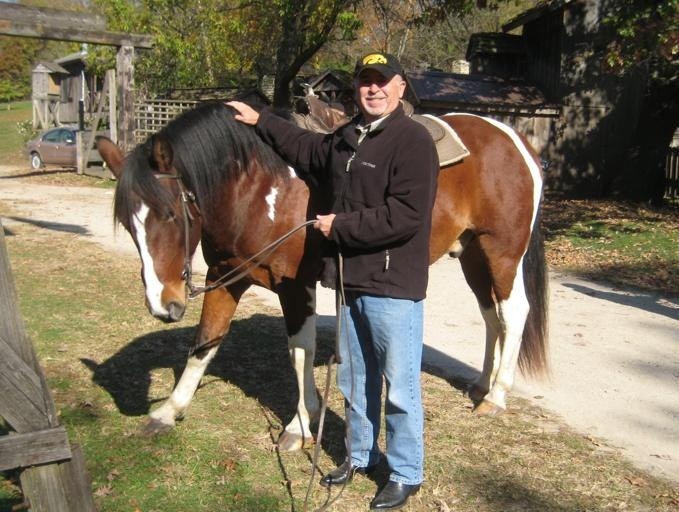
[224,52,439,510]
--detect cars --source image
[26,125,92,170]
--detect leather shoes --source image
[320,461,375,487]
[370,481,421,510]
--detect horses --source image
[94,98,552,454]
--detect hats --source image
[355,52,401,81]
